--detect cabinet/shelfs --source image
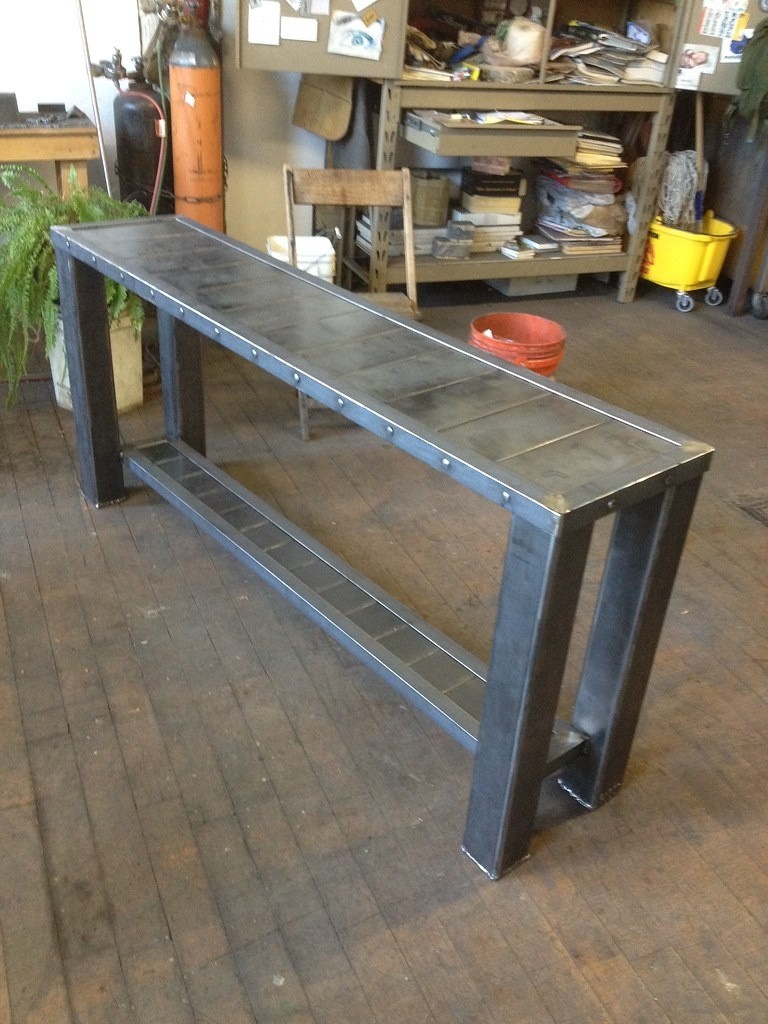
[49,215,715,880]
[236,0,768,304]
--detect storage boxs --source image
[486,274,578,297]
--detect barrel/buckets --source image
[469,312,568,378]
[266,235,336,283]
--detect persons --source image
[681,49,708,68]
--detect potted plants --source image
[0,160,150,413]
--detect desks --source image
[1,110,100,200]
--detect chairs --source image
[282,163,424,443]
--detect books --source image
[352,214,449,258]
[443,163,528,253]
[545,20,668,89]
[501,128,629,259]
[402,65,458,84]
